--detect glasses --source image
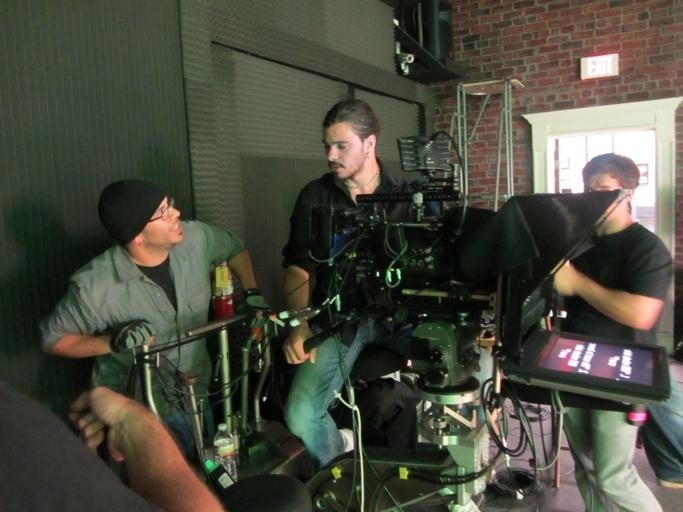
[149,197,175,224]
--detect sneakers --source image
[339,428,355,453]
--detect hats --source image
[98,179,167,244]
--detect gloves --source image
[110,319,156,354]
[237,288,271,328]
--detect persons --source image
[549,153,673,512]
[0,383,227,512]
[279,99,451,467]
[36,177,271,473]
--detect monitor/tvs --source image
[516,329,672,406]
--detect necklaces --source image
[344,162,380,189]
